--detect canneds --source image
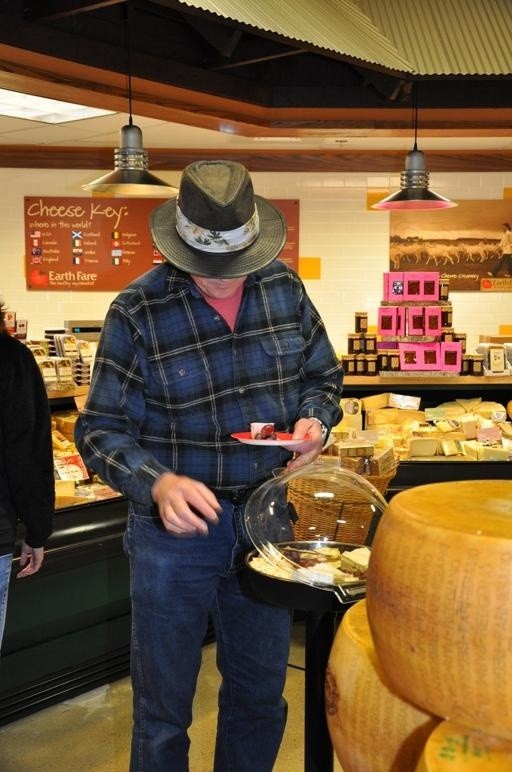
[341,311,400,376]
[441,306,484,375]
[439,279,451,301]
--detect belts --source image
[197,479,262,502]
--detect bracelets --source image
[308,416,328,447]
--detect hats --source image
[148,159,288,280]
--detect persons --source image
[487,223,512,278]
[71,160,345,771]
[0,302,56,650]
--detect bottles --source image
[340,273,484,373]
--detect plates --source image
[231,431,312,448]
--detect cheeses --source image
[50,409,88,507]
[318,392,512,477]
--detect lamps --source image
[370,79,458,210]
[80,7,180,192]
